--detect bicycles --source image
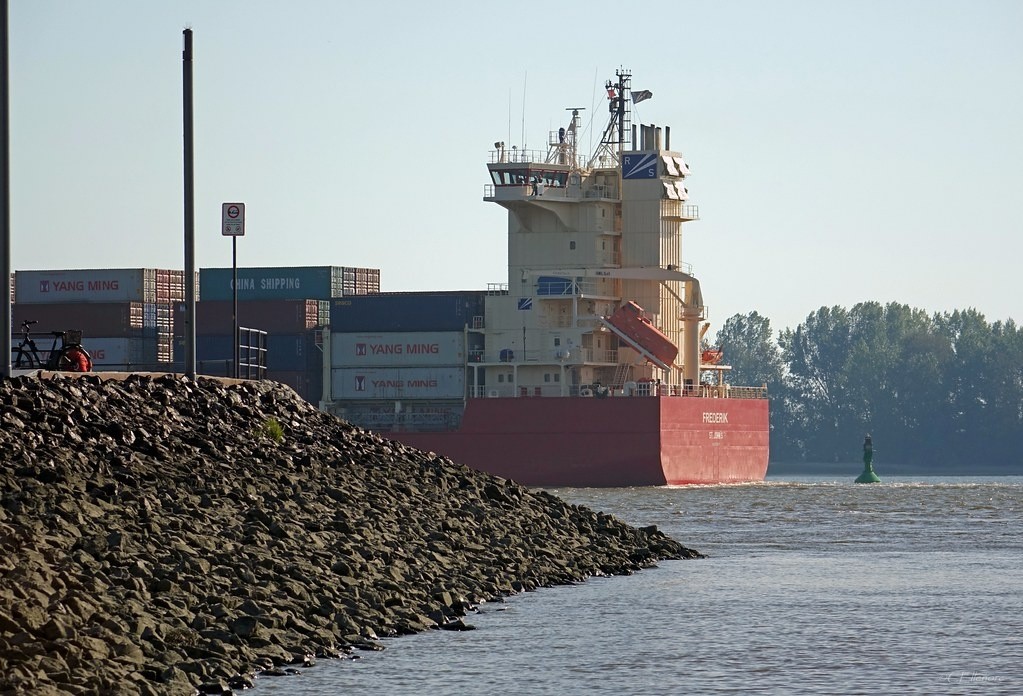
[11,318,93,372]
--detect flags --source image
[631,90,653,104]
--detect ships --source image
[10,66,770,487]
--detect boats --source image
[702,350,724,363]
[605,300,679,371]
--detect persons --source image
[593,378,601,385]
[533,175,542,195]
